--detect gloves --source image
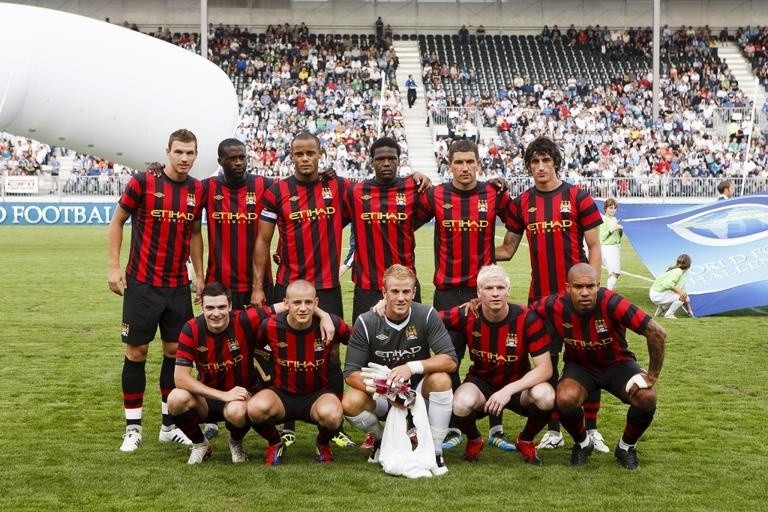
[360,363,416,407]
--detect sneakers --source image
[488,430,517,450]
[263,430,297,466]
[570,428,610,467]
[464,433,484,461]
[313,432,382,464]
[654,304,678,319]
[229,436,249,464]
[158,423,218,464]
[613,441,638,469]
[410,425,464,449]
[515,432,544,465]
[120,425,143,451]
[537,432,564,450]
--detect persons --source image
[2,14,767,198]
[108,126,671,470]
[648,254,696,319]
[716,179,736,202]
